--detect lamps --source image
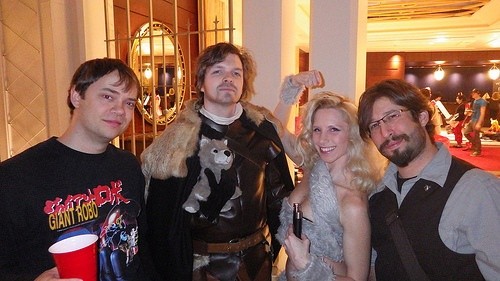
[143,63,152,80]
[487,59,500,81]
[431,60,446,81]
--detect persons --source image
[140,43,295,281]
[0,58,146,281]
[357,79,500,281]
[273,70,388,281]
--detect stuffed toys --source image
[182,133,243,213]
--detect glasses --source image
[367,107,409,135]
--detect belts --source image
[193,224,269,253]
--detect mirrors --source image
[129,22,187,127]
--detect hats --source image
[470,88,479,93]
[456,96,462,99]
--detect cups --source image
[48,234,99,281]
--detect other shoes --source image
[453,144,462,148]
[472,151,480,155]
